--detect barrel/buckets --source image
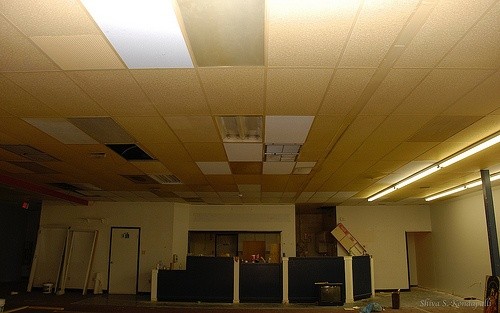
[0,299,5,312]
[43,283,53,293]
